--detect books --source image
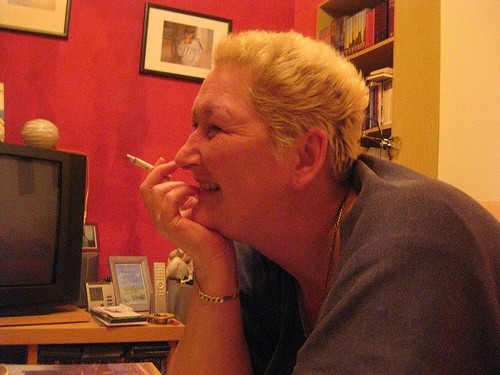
[90,304,147,327]
[319,0,395,57]
[360,67,393,130]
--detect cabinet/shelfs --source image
[315,0,500,222]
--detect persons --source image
[139,30,500,375]
[168,27,203,66]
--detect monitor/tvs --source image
[0,142,88,317]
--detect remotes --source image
[153,262,167,313]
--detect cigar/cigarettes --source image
[126,154,172,179]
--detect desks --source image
[0,307,185,365]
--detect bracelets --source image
[192,271,241,304]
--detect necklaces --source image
[295,195,348,341]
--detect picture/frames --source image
[108,255,154,312]
[81,221,101,252]
[139,2,233,84]
[0,0,72,39]
